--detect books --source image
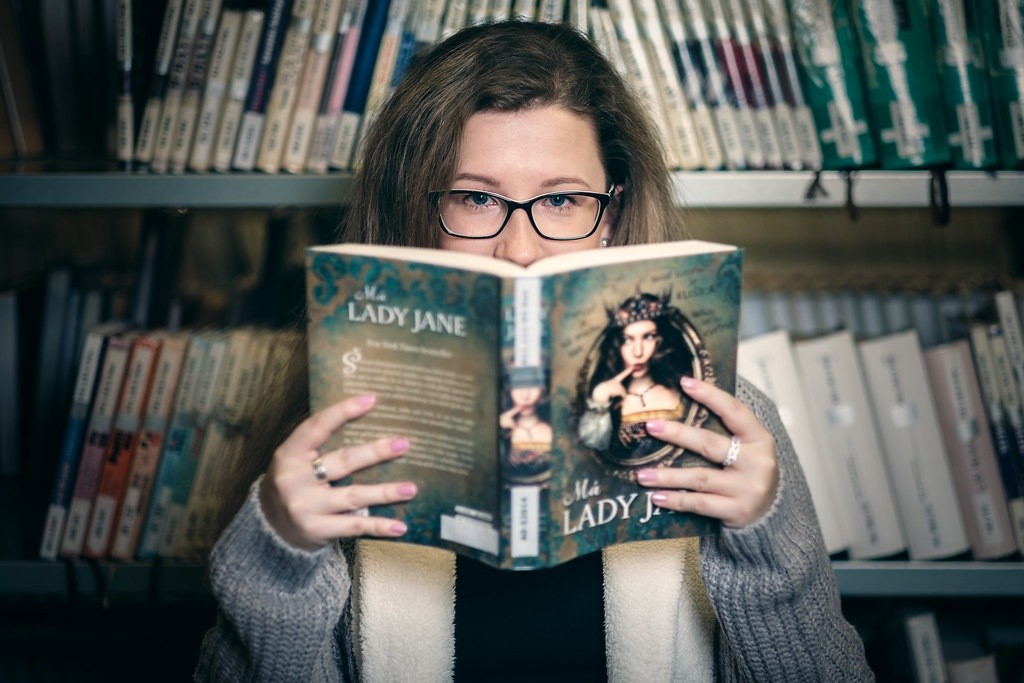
[571,0,1024,683]
[0,0,574,683]
[304,242,743,572]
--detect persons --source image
[193,21,875,683]
[578,294,692,459]
[500,368,553,476]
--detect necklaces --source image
[516,421,542,439]
[627,383,657,408]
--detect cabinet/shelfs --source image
[0,171,1024,593]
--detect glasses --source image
[421,181,616,242]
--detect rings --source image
[311,457,326,480]
[724,435,741,466]
